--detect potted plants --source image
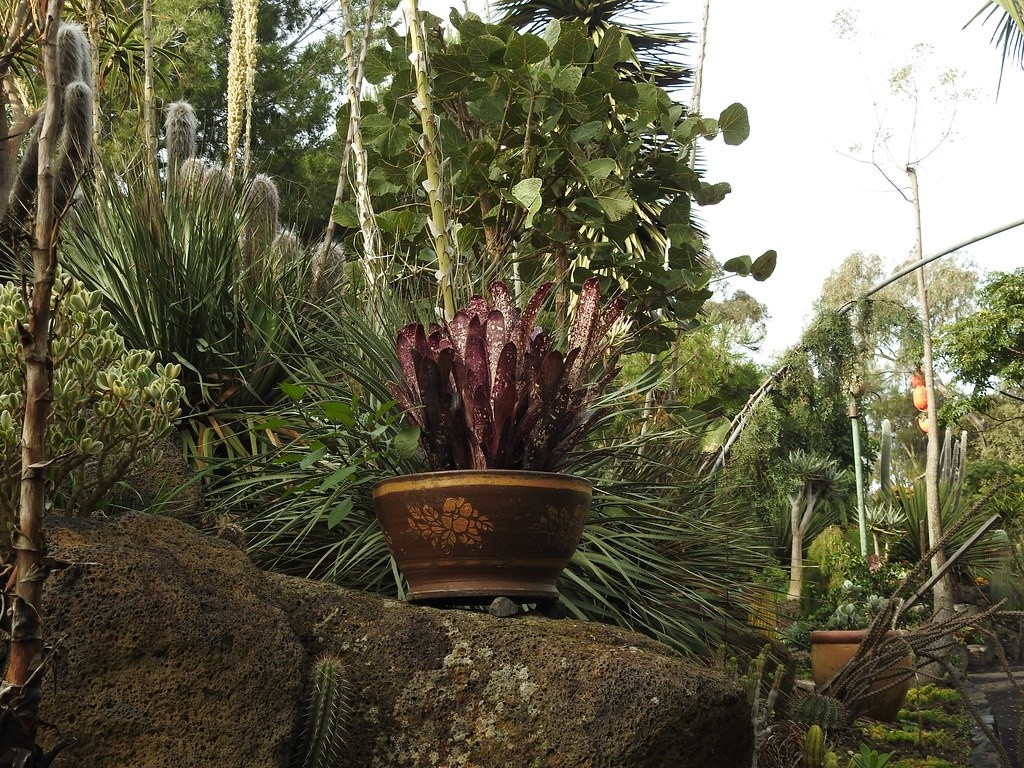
[373,277,624,600]
[808,581,915,721]
